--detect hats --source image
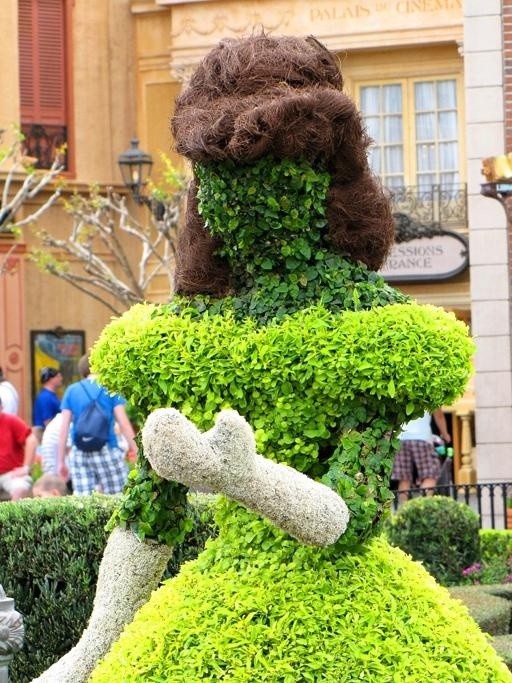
[41,367,56,383]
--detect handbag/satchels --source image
[73,379,109,451]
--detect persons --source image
[38,407,74,496]
[392,403,452,509]
[0,396,40,503]
[0,365,19,414]
[55,351,140,499]
[28,469,68,500]
[30,365,62,445]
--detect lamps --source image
[115,134,164,220]
